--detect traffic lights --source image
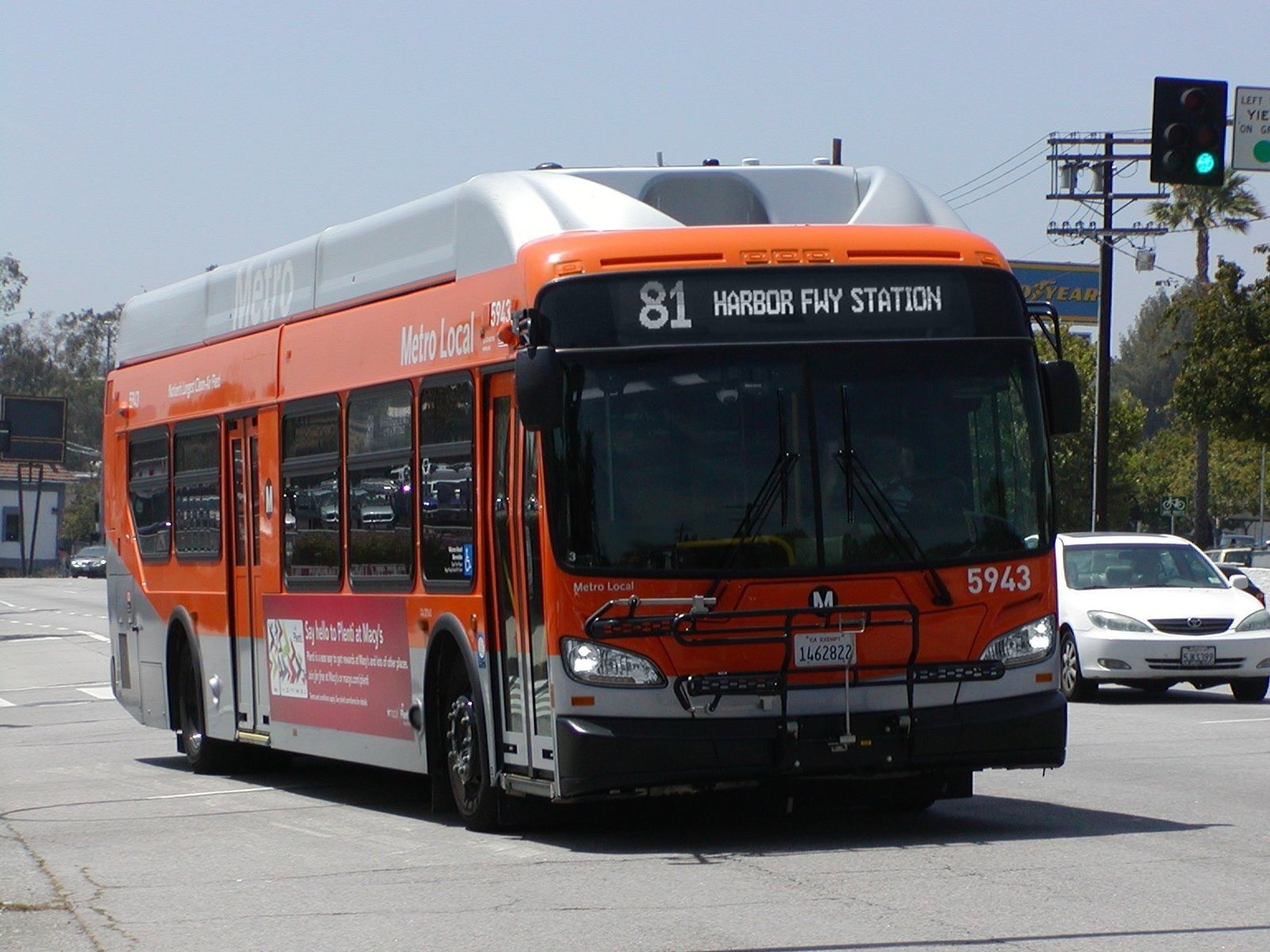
[1149,76,1228,188]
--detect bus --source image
[102,137,1083,834]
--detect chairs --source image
[1104,565,1134,585]
[1138,569,1167,586]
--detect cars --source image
[1024,531,1270,702]
[1162,533,1270,608]
[279,461,540,540]
[69,544,108,578]
